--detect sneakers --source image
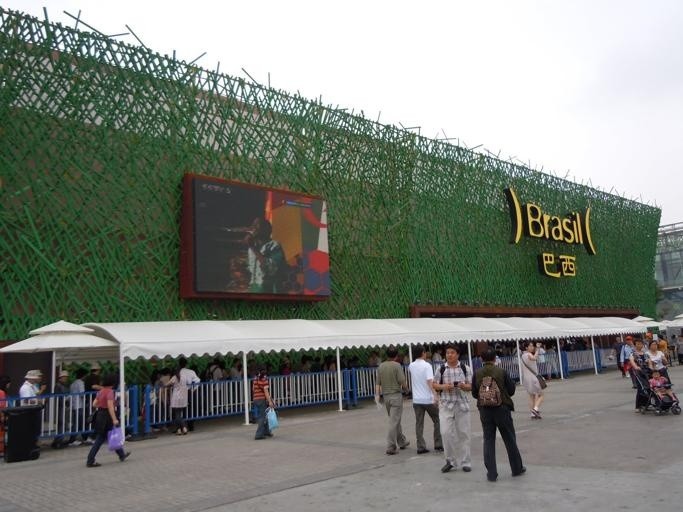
[52,424,194,467]
[531,408,542,419]
[532,416,538,419]
[387,440,526,481]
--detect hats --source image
[90,360,101,370]
[59,370,70,378]
[24,369,42,379]
[625,336,632,340]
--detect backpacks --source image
[202,365,220,382]
[477,367,503,408]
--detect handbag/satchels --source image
[268,407,279,429]
[536,374,547,389]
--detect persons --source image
[151,356,200,436]
[0,362,122,445]
[615,333,682,412]
[409,346,446,453]
[240,217,287,293]
[432,347,474,473]
[85,375,132,468]
[519,338,570,418]
[205,352,375,440]
[375,346,411,454]
[472,347,526,482]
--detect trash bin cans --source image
[2,405,45,463]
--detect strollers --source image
[631,364,681,415]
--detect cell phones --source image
[454,381,459,387]
